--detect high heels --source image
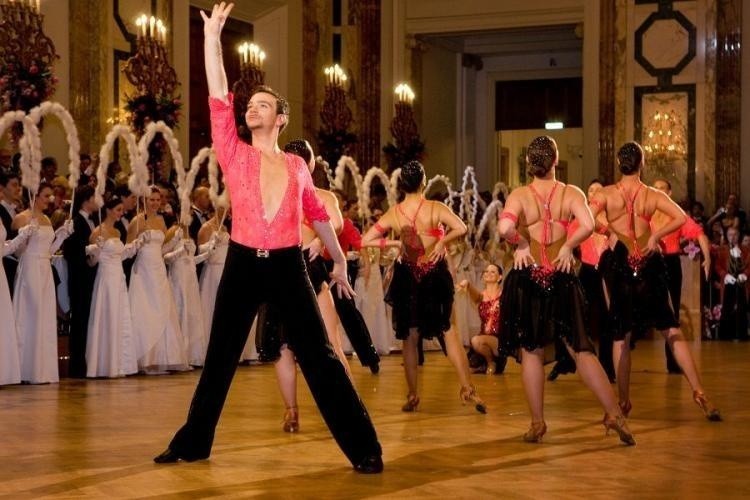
[524,420,547,442]
[620,400,632,414]
[402,394,420,412]
[460,386,490,414]
[283,406,301,432]
[693,389,723,421]
[604,412,636,445]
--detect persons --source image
[709,193,749,339]
[152,1,385,475]
[454,264,504,375]
[498,137,638,444]
[360,163,486,415]
[252,134,352,434]
[2,136,519,400]
[582,142,724,421]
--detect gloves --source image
[211,231,223,244]
[182,239,194,252]
[209,245,219,255]
[131,238,142,252]
[94,234,105,247]
[85,244,100,256]
[174,226,187,241]
[56,222,73,241]
[141,229,153,244]
[16,223,40,240]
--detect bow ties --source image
[123,212,128,219]
[200,212,209,217]
[87,214,95,220]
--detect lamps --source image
[320,64,353,132]
[390,84,419,149]
[122,14,182,100]
[231,42,266,112]
[0,0,56,68]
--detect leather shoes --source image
[471,363,685,385]
[152,446,175,464]
[357,455,384,472]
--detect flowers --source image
[313,132,357,172]
[383,142,428,170]
[0,56,59,115]
[121,95,185,140]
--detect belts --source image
[225,238,303,259]
